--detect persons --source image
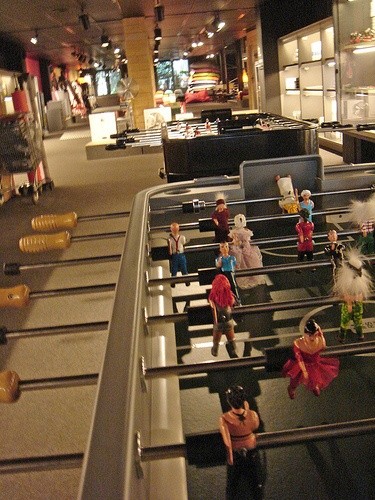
[167,222,190,287]
[274,174,299,215]
[324,229,346,277]
[173,113,272,136]
[215,240,243,308]
[357,223,375,256]
[297,189,315,226]
[335,247,374,343]
[282,320,340,399]
[227,213,273,290]
[211,192,231,242]
[208,274,240,358]
[218,384,263,494]
[295,208,317,273]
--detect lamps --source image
[77,7,93,31]
[205,29,214,39]
[113,43,129,67]
[153,45,159,53]
[216,17,226,28]
[100,32,110,48]
[191,40,198,49]
[155,40,160,45]
[71,46,102,70]
[154,4,164,24]
[154,28,162,41]
[183,46,192,59]
[213,25,222,32]
[153,53,158,66]
[28,31,39,46]
[197,38,204,47]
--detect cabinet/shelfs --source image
[277,0,375,152]
